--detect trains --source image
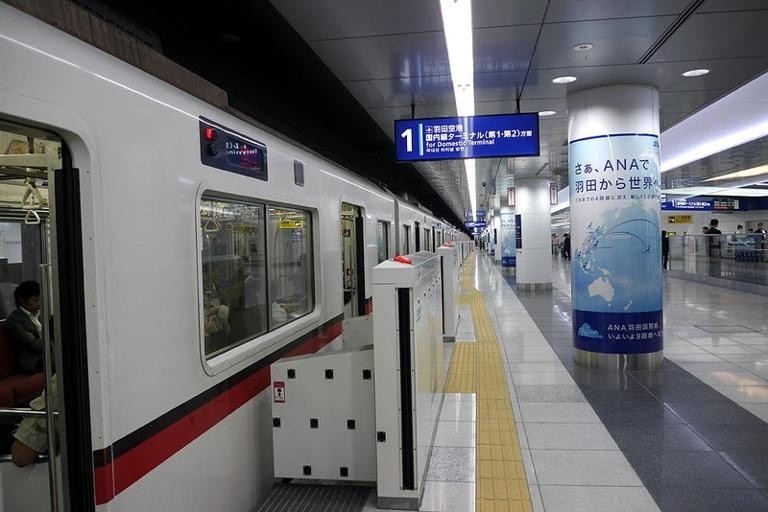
[0,2,468,512]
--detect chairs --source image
[0,321,54,411]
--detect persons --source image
[705,219,723,277]
[194,212,316,363]
[661,230,669,271]
[745,228,754,242]
[734,225,745,243]
[0,278,55,378]
[754,222,768,263]
[475,238,486,250]
[552,232,571,261]
[702,225,709,234]
[9,372,62,469]
